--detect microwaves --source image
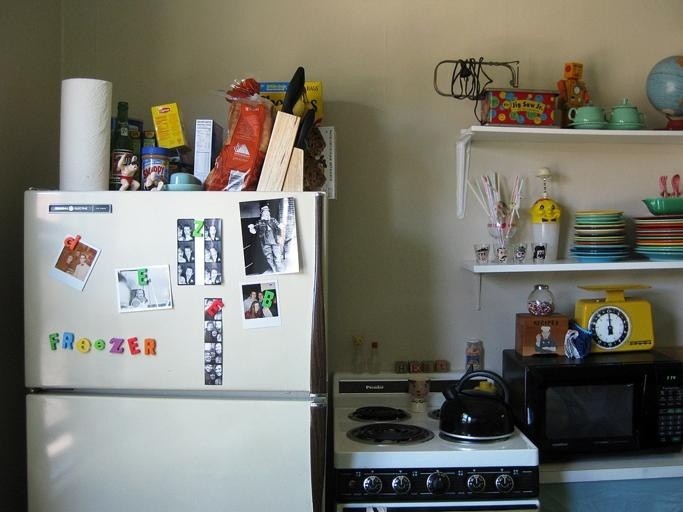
[502,348,683,463]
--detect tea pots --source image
[439,369,514,443]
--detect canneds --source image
[142,146,170,191]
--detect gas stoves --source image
[334,405,539,502]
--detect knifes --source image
[294,108,316,148]
[281,66,305,115]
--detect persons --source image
[204,242,215,262]
[177,223,185,241]
[130,288,147,307]
[180,266,195,284]
[210,247,221,262]
[142,170,167,191]
[209,225,220,241]
[54,248,90,281]
[115,152,141,190]
[204,268,213,285]
[210,269,221,285]
[203,297,223,385]
[178,264,186,284]
[177,246,188,263]
[184,246,194,263]
[243,291,278,319]
[118,273,134,310]
[533,326,557,356]
[183,224,194,241]
[247,205,285,274]
[204,224,212,242]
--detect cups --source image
[407,377,431,414]
[568,100,605,122]
[608,98,647,124]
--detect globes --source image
[644,54,683,130]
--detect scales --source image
[574,283,656,354]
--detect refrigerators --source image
[22,190,329,512]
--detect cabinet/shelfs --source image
[455,124,683,313]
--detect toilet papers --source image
[58,77,113,192]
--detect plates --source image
[568,210,631,262]
[567,122,609,129]
[608,121,648,130]
[633,216,683,262]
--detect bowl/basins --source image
[642,198,683,216]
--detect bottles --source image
[108,101,134,190]
[527,284,555,316]
[465,339,481,371]
[369,342,380,373]
